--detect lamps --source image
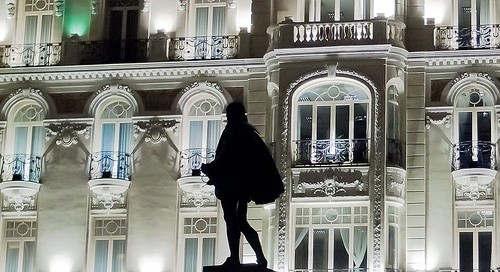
[70,33,79,41]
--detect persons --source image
[201,101,285,272]
